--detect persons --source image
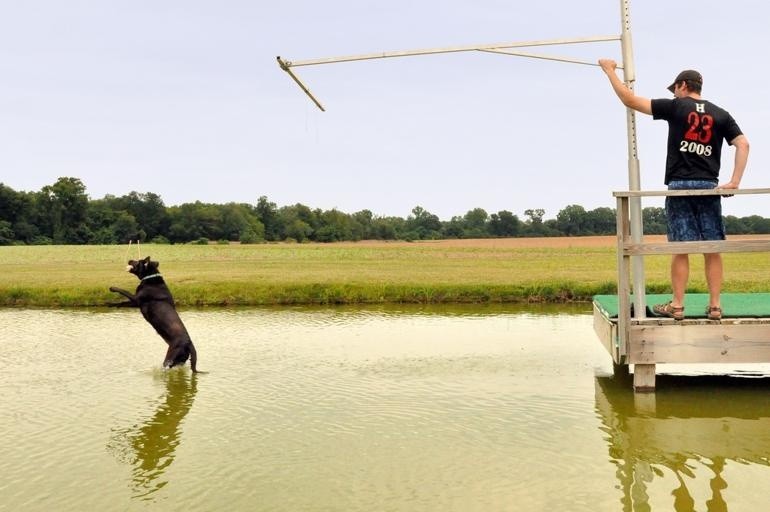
[599,59,749,318]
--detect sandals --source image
[705,305,722,320]
[653,301,684,320]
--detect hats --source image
[668,70,703,93]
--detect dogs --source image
[104,255,197,372]
